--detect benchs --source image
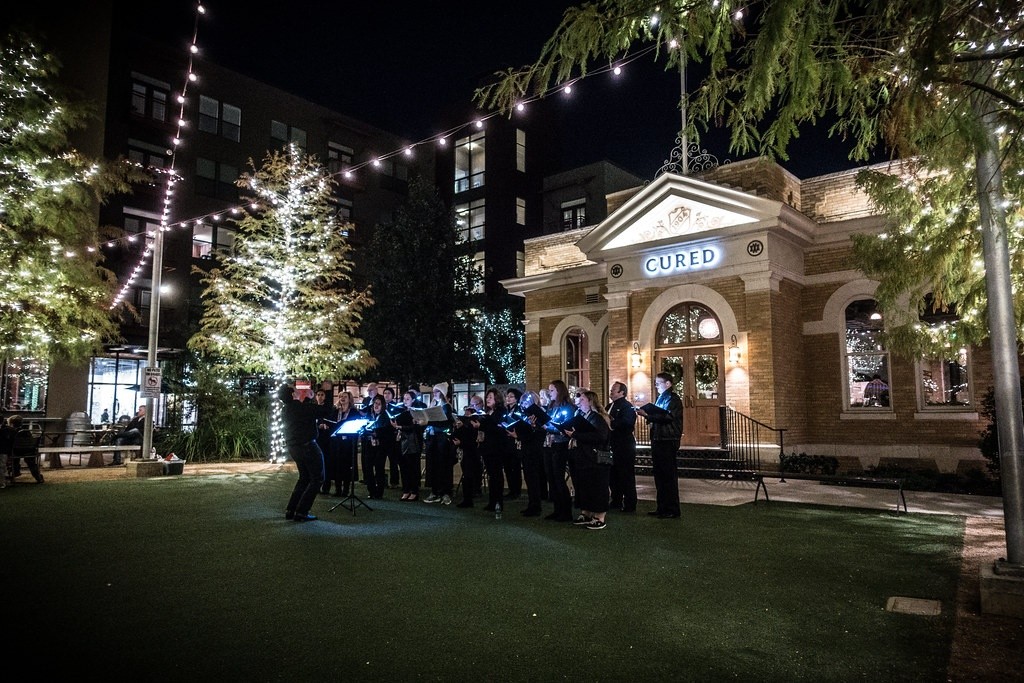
[37,444,141,469]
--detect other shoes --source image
[648,506,664,515]
[609,501,623,511]
[319,485,573,523]
[658,511,681,520]
[286,511,317,522]
[620,507,635,514]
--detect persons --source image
[329,380,657,537]
[278,384,341,521]
[108,405,146,466]
[100,408,109,423]
[0,414,44,488]
[637,373,684,518]
[863,375,887,399]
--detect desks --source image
[32,428,119,466]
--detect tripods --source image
[328,419,375,517]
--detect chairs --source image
[7,429,45,485]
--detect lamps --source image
[630,342,643,368]
[728,334,742,364]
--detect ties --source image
[608,402,615,415]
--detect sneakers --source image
[587,516,606,530]
[572,512,591,524]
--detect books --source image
[500,419,532,433]
[550,414,596,437]
[385,409,408,426]
[408,405,448,422]
[456,414,480,427]
[523,403,552,426]
[631,403,670,415]
[335,418,369,434]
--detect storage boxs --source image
[156,459,185,475]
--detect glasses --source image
[655,381,666,386]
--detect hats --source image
[433,382,449,396]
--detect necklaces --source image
[587,410,591,414]
